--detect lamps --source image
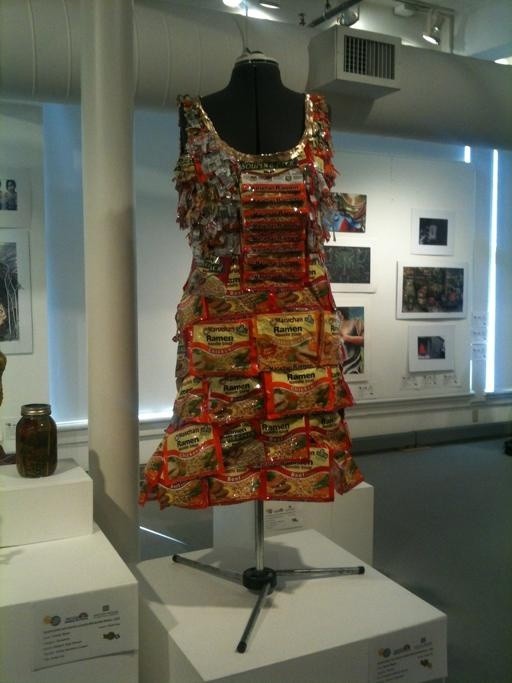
[422,8,447,45]
[222,0,248,11]
